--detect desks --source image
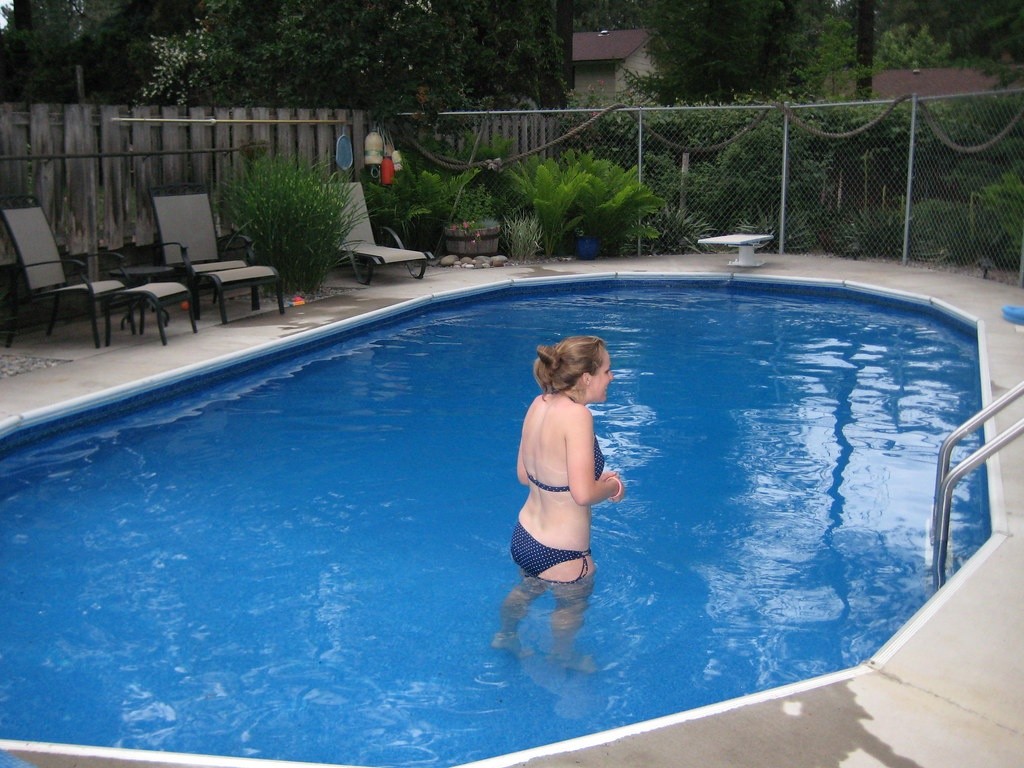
[109,266,175,334]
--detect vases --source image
[444,225,500,258]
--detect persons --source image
[512,335,623,585]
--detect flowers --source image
[450,220,481,249]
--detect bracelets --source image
[606,477,622,499]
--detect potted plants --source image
[559,148,666,261]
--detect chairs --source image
[148,182,285,325]
[322,182,435,286]
[0,194,136,349]
